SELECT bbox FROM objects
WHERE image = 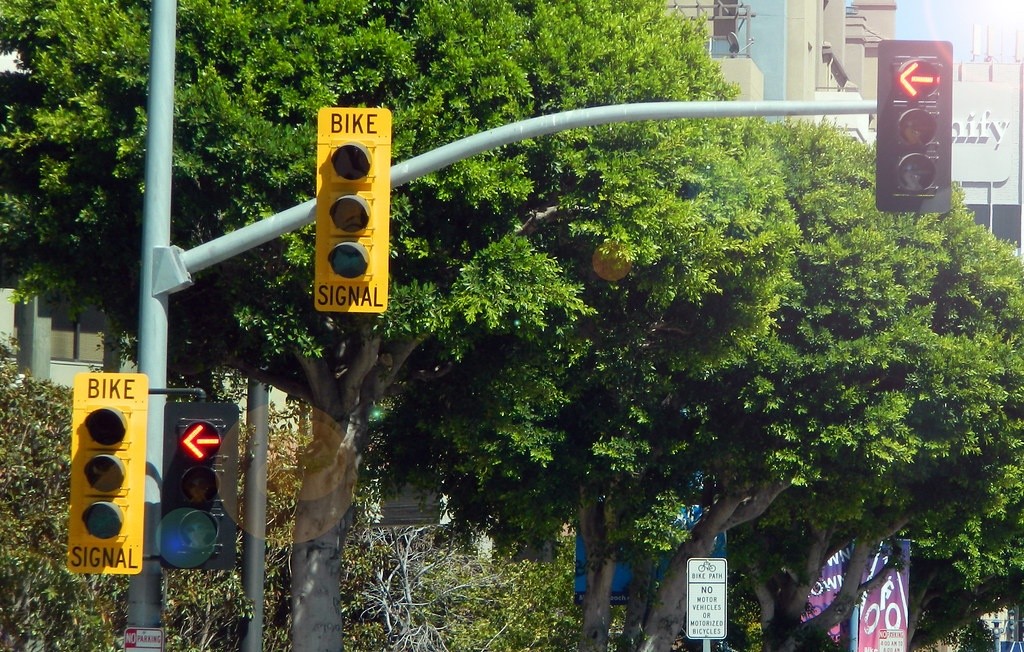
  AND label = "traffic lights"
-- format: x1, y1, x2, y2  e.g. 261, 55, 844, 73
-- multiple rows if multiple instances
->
314, 107, 393, 315
876, 39, 951, 211
66, 372, 143, 576
161, 400, 239, 569
